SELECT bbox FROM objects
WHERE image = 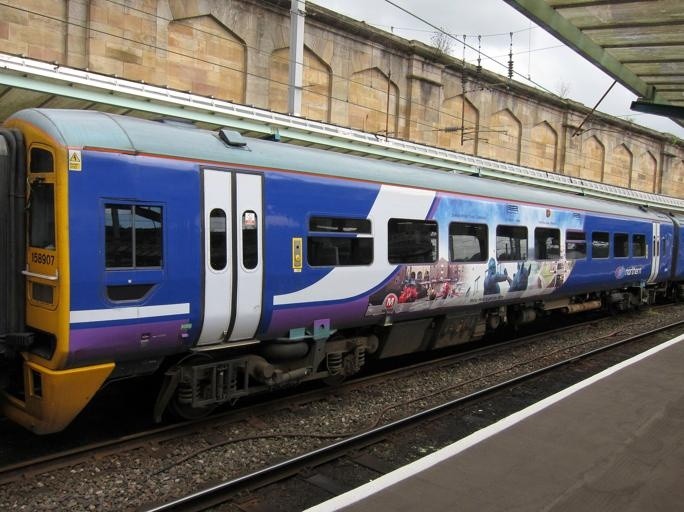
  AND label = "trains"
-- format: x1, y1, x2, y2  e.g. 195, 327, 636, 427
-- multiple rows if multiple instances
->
1, 107, 684, 439
